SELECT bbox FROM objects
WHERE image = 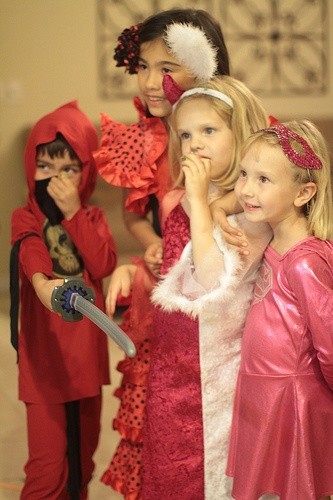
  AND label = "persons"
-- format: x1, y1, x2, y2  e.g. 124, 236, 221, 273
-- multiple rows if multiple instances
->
225, 120, 333, 500
105, 75, 273, 500
92, 8, 250, 500
9, 100, 117, 500
